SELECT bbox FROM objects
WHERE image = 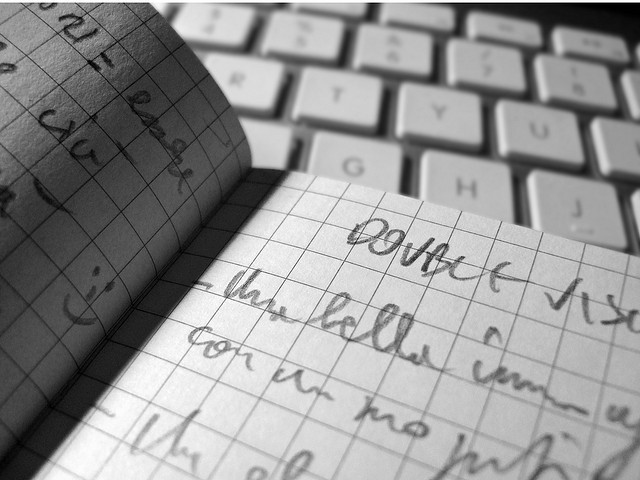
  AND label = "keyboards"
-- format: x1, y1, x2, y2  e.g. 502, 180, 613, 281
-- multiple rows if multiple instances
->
141, 3, 639, 256
